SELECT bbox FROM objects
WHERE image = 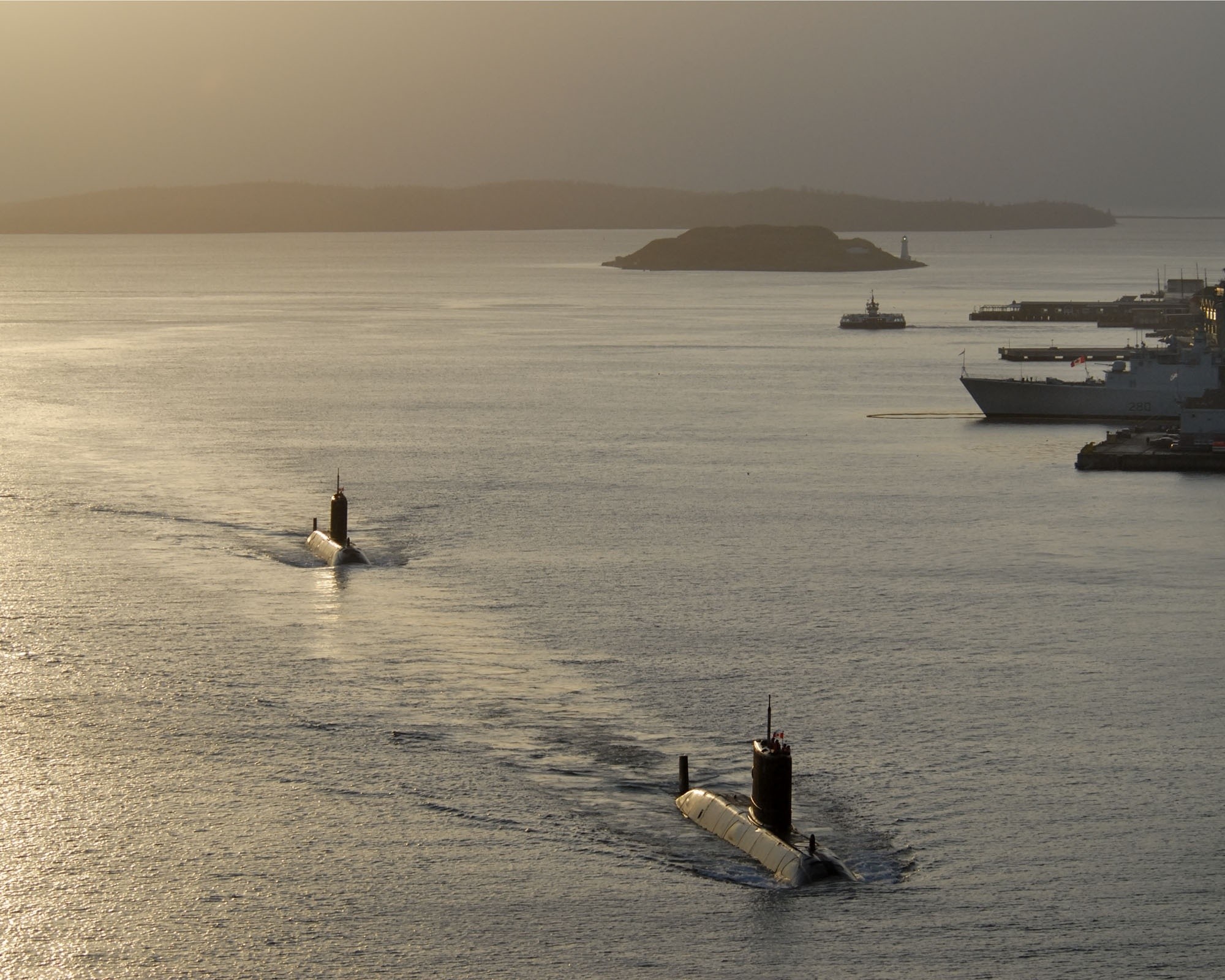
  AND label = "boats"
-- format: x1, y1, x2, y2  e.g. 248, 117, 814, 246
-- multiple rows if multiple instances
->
959, 268, 1225, 419
